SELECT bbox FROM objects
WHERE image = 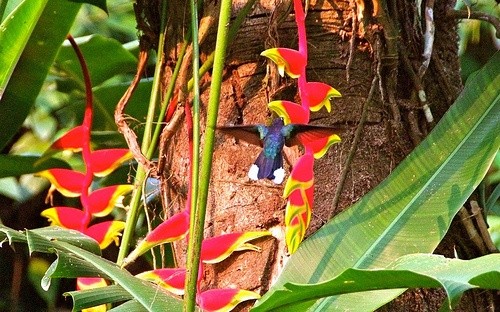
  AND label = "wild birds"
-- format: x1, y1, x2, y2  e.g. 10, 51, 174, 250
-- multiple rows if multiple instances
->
207, 111, 347, 183
